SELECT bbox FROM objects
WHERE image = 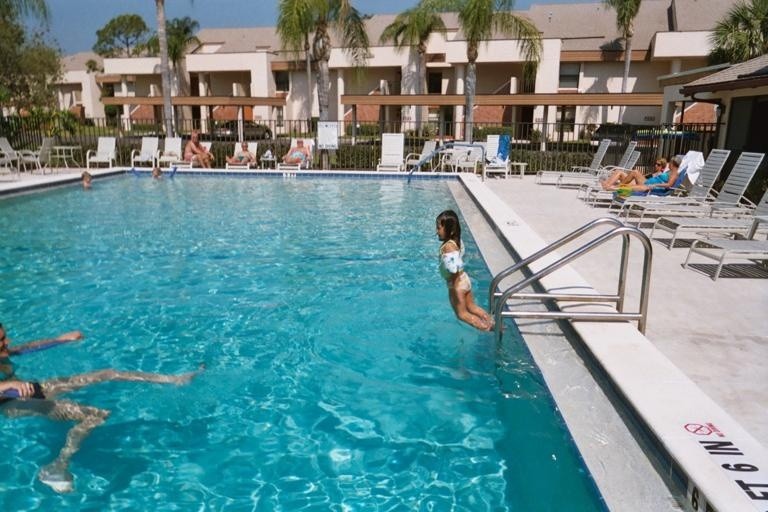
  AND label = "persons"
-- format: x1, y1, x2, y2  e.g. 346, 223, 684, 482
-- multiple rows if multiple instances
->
225, 141, 257, 166
152, 168, 162, 179
81, 171, 93, 188
434, 208, 495, 336
0, 323, 205, 483
282, 139, 310, 163
623, 157, 682, 193
603, 158, 667, 190
184, 133, 215, 168
